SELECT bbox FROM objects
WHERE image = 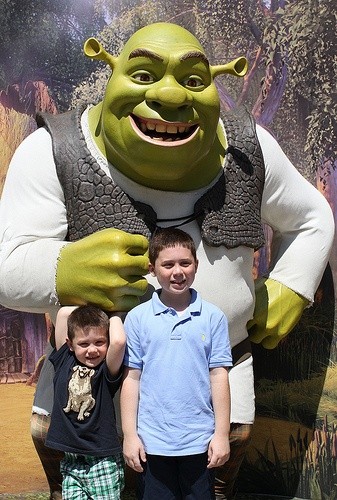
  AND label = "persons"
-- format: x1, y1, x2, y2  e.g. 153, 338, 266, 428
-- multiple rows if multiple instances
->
0, 22, 335, 500
44, 305, 127, 500
120, 228, 233, 500
